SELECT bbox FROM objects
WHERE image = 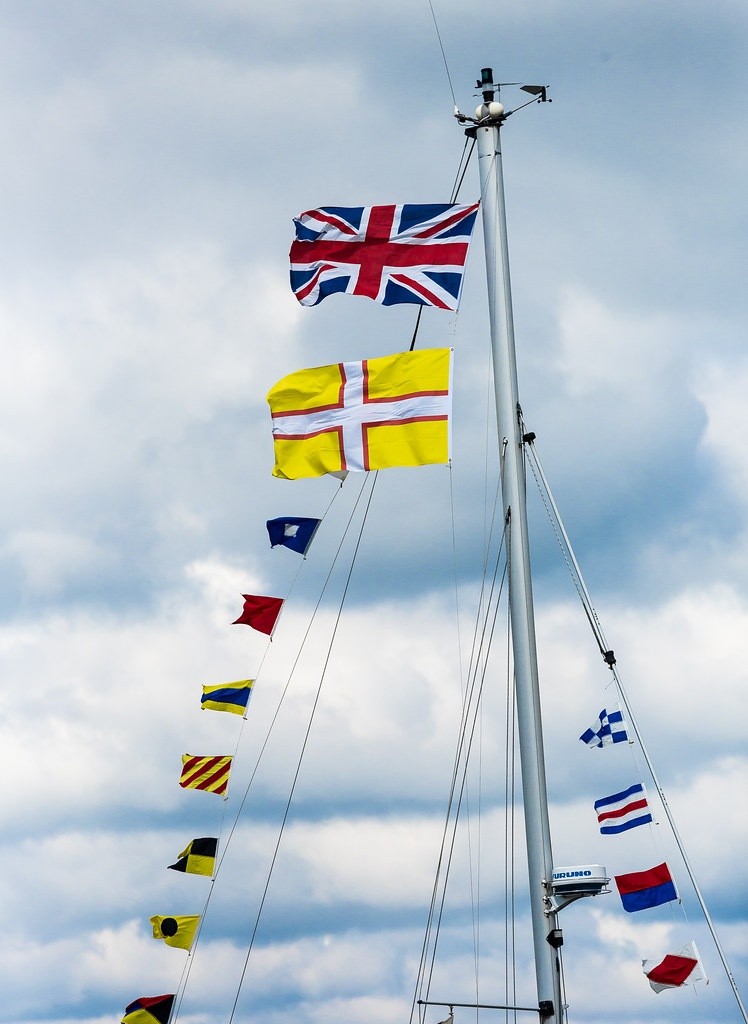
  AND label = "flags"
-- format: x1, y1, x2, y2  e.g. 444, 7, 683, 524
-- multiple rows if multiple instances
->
119, 994, 176, 1024
593, 782, 652, 835
179, 752, 234, 796
290, 204, 478, 313
268, 516, 321, 559
577, 701, 631, 751
201, 678, 255, 718
149, 914, 203, 952
614, 862, 679, 913
266, 346, 456, 482
167, 837, 219, 878
231, 591, 286, 642
641, 941, 708, 994
